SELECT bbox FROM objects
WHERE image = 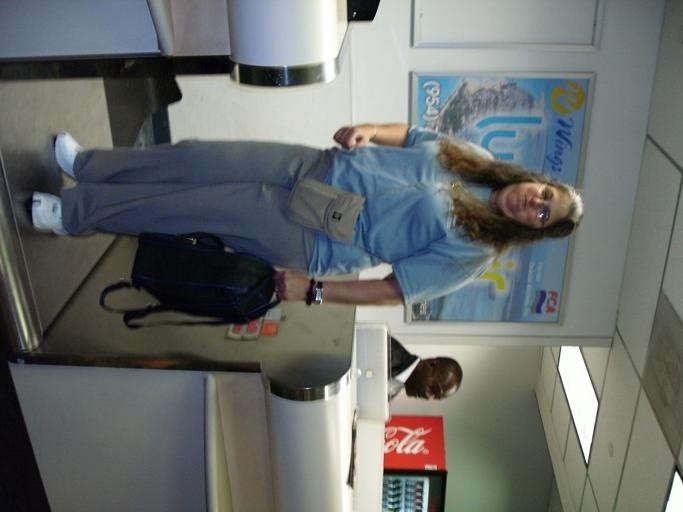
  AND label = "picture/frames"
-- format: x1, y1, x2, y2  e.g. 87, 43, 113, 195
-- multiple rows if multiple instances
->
403, 66, 598, 328
